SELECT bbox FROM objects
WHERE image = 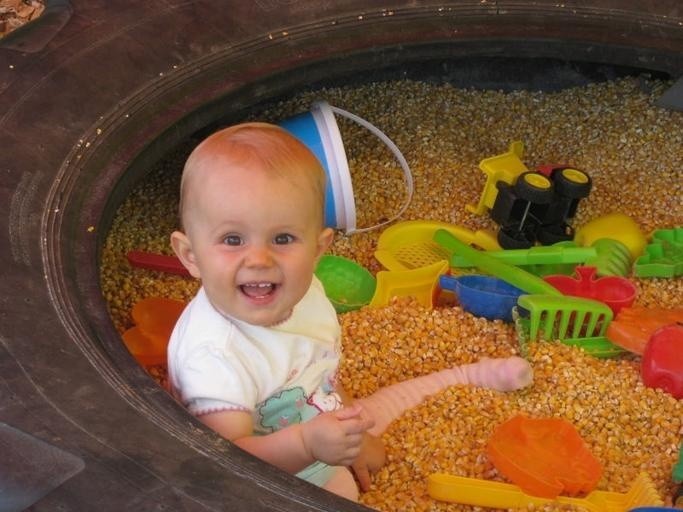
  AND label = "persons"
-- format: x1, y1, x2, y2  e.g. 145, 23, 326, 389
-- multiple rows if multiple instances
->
166, 122, 535, 503
